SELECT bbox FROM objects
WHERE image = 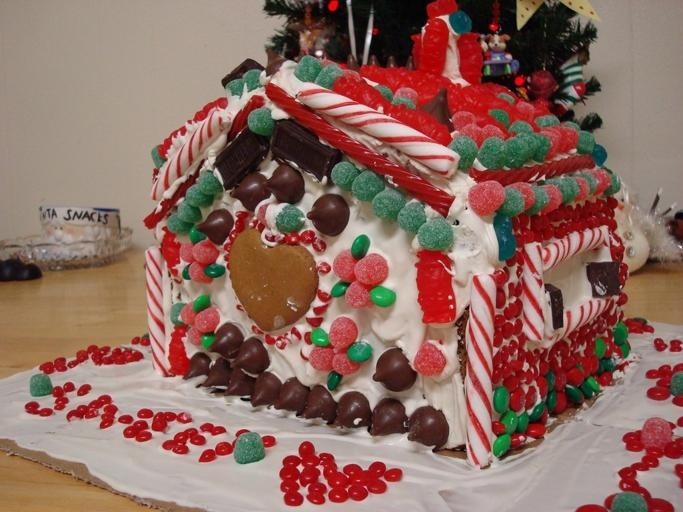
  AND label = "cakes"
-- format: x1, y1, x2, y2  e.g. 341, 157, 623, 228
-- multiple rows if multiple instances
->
0, 0, 683, 512
